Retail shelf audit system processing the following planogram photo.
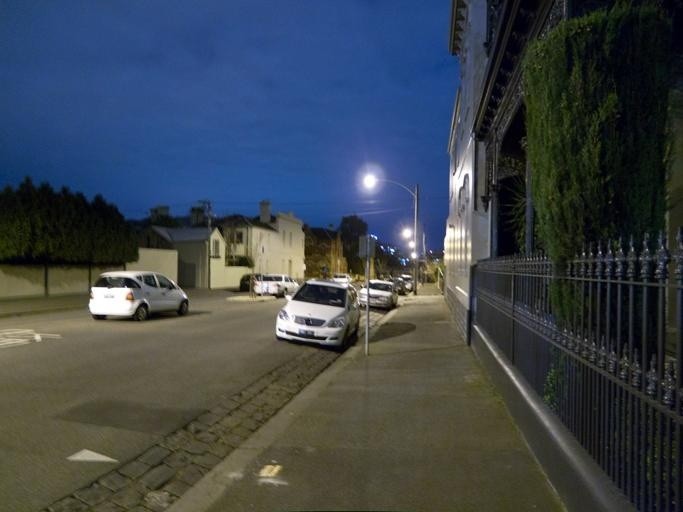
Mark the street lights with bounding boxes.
[364,173,419,294]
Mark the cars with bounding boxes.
[254,272,415,350]
[86,271,189,322]
